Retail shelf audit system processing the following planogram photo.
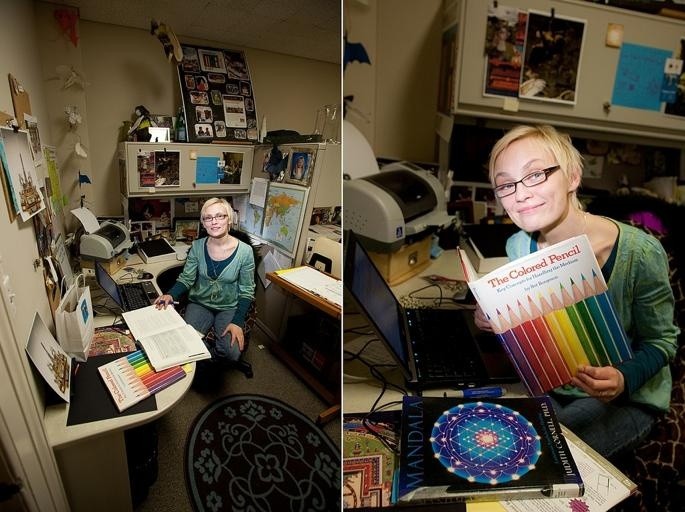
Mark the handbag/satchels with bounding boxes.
[55,274,96,363]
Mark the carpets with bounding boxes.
[182,393,341,512]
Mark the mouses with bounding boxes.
[451,289,476,305]
[137,272,154,280]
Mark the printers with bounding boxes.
[343,159,455,253]
[79,220,132,260]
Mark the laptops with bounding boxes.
[346,229,521,390]
[94,262,160,312]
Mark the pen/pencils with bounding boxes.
[443,386,508,397]
[158,300,180,305]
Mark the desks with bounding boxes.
[344,249,642,510]
[41,243,199,512]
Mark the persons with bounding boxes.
[292,155,305,180]
[191,92,205,104]
[197,126,205,136]
[205,128,211,136]
[139,158,152,173]
[488,25,514,62]
[196,110,205,122]
[154,196,258,379]
[127,104,159,142]
[486,122,681,458]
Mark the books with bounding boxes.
[120,303,212,373]
[456,234,636,398]
[397,394,585,505]
[96,349,186,414]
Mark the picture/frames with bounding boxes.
[148,126,171,143]
[284,146,317,186]
[171,215,202,241]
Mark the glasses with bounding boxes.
[494,166,560,199]
[202,214,228,221]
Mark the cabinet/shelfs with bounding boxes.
[241,143,341,352]
[259,265,344,426]
[436,0,685,144]
[119,140,255,198]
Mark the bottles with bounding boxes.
[175,105,186,142]
[260,114,267,144]
[307,104,339,144]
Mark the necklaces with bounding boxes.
[541,213,588,248]
[208,256,220,276]
